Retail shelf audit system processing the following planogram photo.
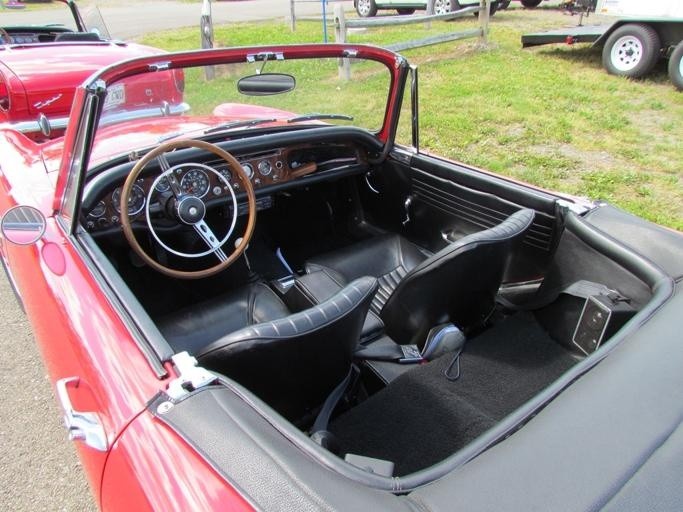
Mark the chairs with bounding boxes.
[147,273,379,416]
[302,206,538,354]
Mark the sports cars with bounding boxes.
[2,0,192,146]
[1,41,681,512]
